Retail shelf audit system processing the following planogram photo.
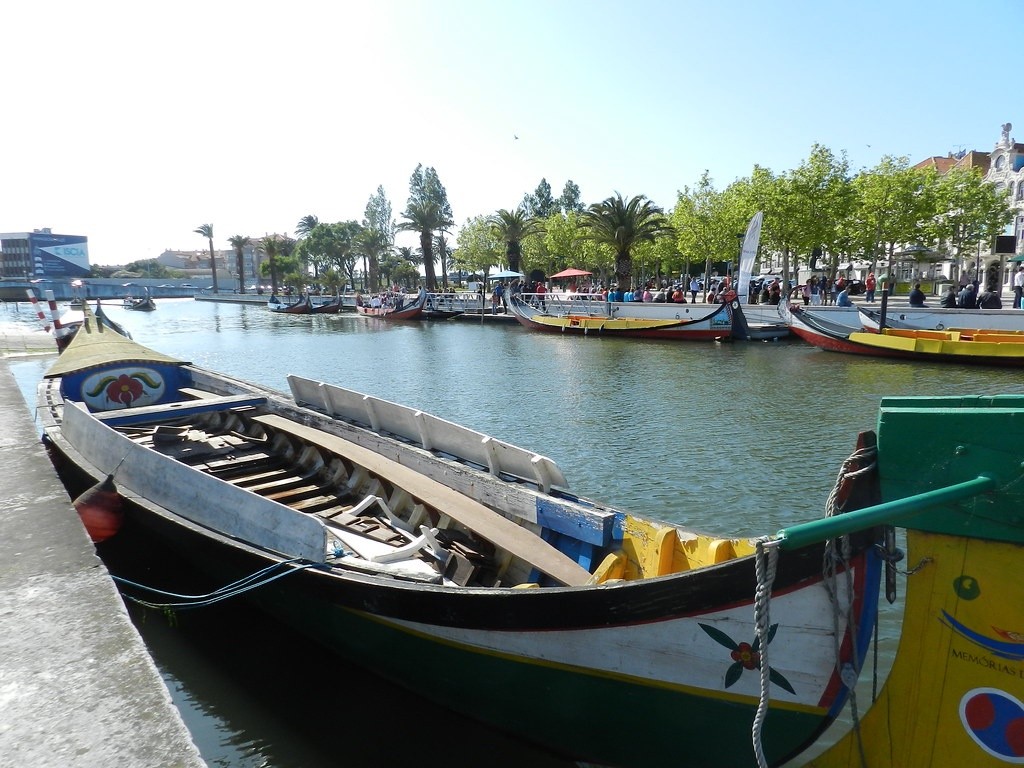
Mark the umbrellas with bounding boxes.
[487,269,524,285]
[550,267,593,280]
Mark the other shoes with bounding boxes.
[829,303,832,306]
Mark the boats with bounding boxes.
[504,287,751,349]
[855,305,1024,335]
[356,287,427,321]
[774,298,1024,363]
[31,300,1023,765]
[123,286,158,312]
[264,294,313,314]
[311,292,343,314]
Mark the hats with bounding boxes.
[966,284,974,289]
[869,273,875,277]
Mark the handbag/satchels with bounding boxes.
[708,294,714,301]
[800,287,807,291]
[683,299,687,303]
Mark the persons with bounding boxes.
[707,275,854,307]
[476,279,548,315]
[865,273,876,302]
[888,273,897,296]
[1012,261,1024,308]
[941,270,1002,309]
[909,283,929,308]
[357,284,455,309]
[561,274,699,304]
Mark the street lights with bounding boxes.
[546,257,559,289]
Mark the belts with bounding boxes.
[759,300,768,303]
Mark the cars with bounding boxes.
[124,282,139,287]
[30,278,52,283]
[159,283,193,288]
